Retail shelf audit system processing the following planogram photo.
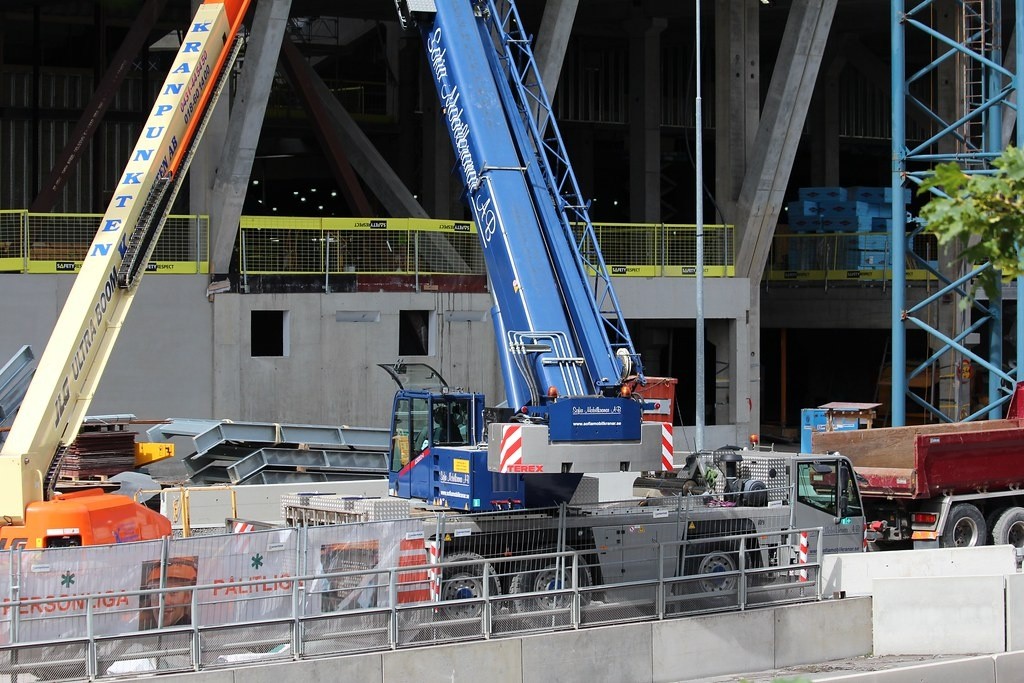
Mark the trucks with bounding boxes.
[810,376,1023,568]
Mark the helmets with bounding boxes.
[145,556,197,585]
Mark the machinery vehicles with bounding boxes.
[280,1,792,618]
[1,1,336,679]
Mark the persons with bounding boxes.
[138,556,197,630]
[434,406,465,448]
